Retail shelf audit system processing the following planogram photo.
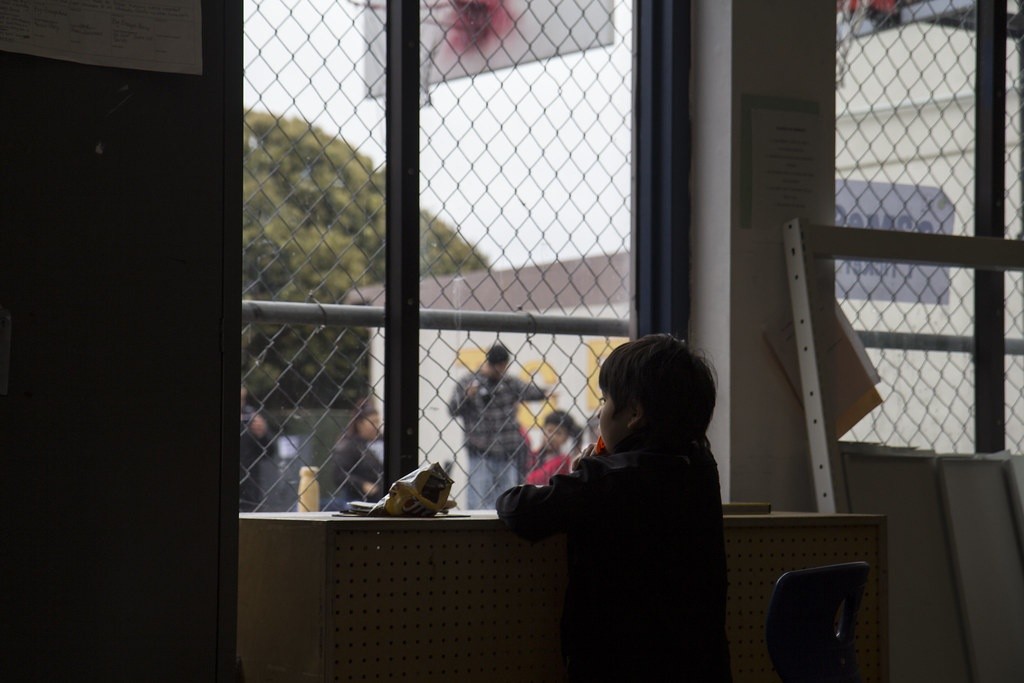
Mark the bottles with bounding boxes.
[296,467,319,512]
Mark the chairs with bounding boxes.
[764,560,871,683]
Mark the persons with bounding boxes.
[239,376,278,512]
[449,345,585,507]
[494,334,734,683]
[332,407,384,502]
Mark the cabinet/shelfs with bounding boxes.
[238,510,891,683]
[0,0,244,683]
